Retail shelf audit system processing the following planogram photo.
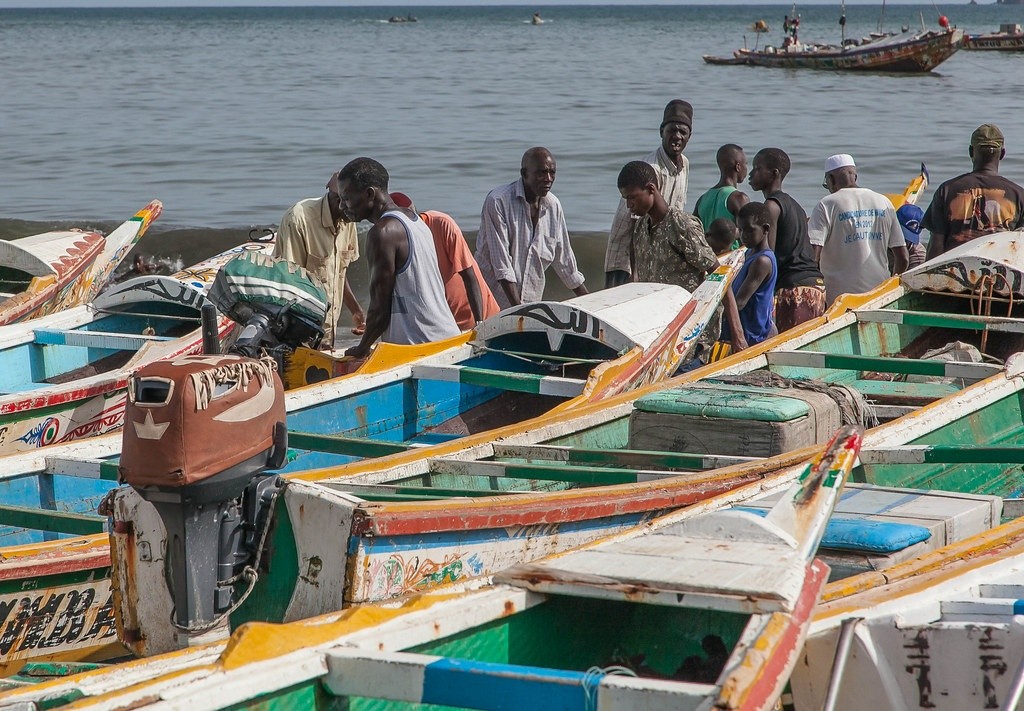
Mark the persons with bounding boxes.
[389,193,500,331]
[732,203,777,345]
[339,157,462,361]
[617,160,749,374]
[474,147,588,310]
[749,148,824,332]
[693,144,750,250]
[703,218,740,254]
[533,12,542,23]
[275,173,366,352]
[887,204,926,274]
[920,123,1024,262]
[604,99,692,288]
[809,154,909,310]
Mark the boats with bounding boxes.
[1,196,163,328]
[2,158,1024,711]
[738,0,969,74]
[522,19,553,25]
[389,15,421,25]
[702,53,755,72]
[958,24,1024,53]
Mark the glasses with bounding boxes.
[822,173,833,189]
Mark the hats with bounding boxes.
[388,191,414,210]
[824,153,855,172]
[895,204,926,244]
[661,100,693,132]
[971,123,1004,149]
